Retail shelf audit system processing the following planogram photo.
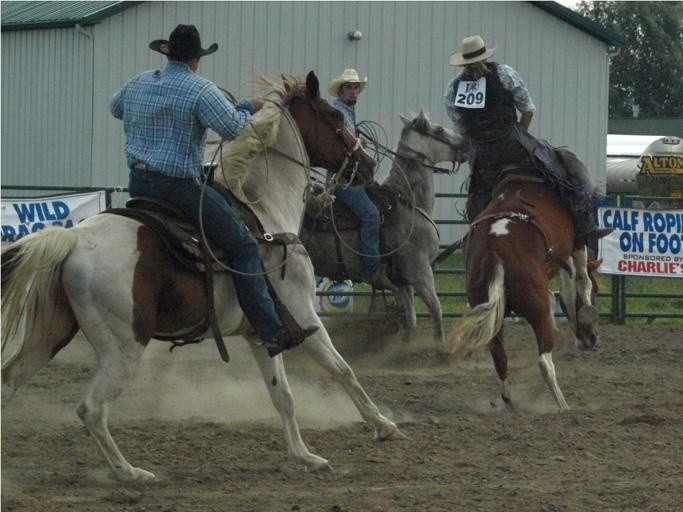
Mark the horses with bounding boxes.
[1,69,410,486]
[456,149,604,411]
[299,106,470,347]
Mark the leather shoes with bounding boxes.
[266,325,318,357]
[369,272,398,291]
[574,228,613,248]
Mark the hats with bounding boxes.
[150,24,218,57]
[448,35,496,66]
[328,69,367,97]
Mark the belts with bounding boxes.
[132,163,154,171]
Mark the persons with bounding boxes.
[107,24,321,359]
[443,34,616,251]
[325,68,399,293]
